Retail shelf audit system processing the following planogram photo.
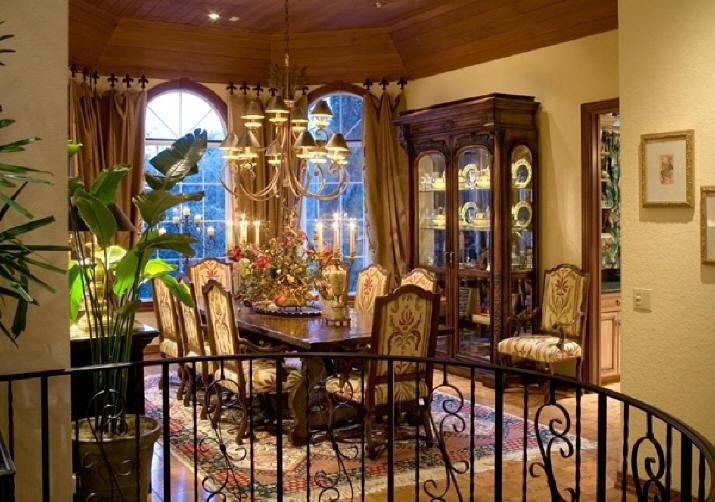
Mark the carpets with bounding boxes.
[143,372,598,500]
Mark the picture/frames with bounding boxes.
[700,185,715,265]
[640,128,695,210]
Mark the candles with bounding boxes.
[158,207,215,237]
[227,221,260,243]
[318,223,355,253]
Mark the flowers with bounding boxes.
[227,206,336,308]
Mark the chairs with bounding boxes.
[186,257,236,296]
[152,273,210,421]
[318,258,441,314]
[495,264,593,407]
[199,279,300,446]
[322,282,441,453]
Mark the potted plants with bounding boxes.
[65,126,209,500]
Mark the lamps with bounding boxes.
[216,1,354,202]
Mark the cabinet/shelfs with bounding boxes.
[392,93,542,390]
[599,124,620,384]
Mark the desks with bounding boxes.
[193,301,379,443]
